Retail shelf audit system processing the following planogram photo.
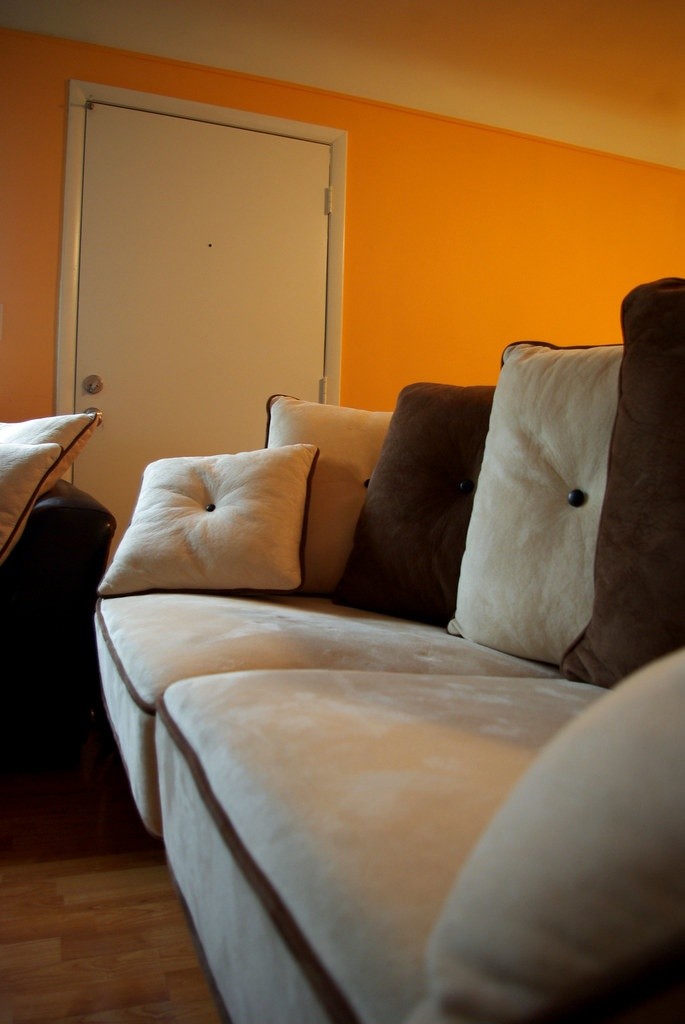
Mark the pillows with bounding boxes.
[265,394,393,598]
[0,413,104,566]
[448,341,624,668]
[333,382,495,627]
[555,276,685,690]
[96,443,321,597]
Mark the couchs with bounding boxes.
[95,597,685,1024]
[0,482,116,776]
